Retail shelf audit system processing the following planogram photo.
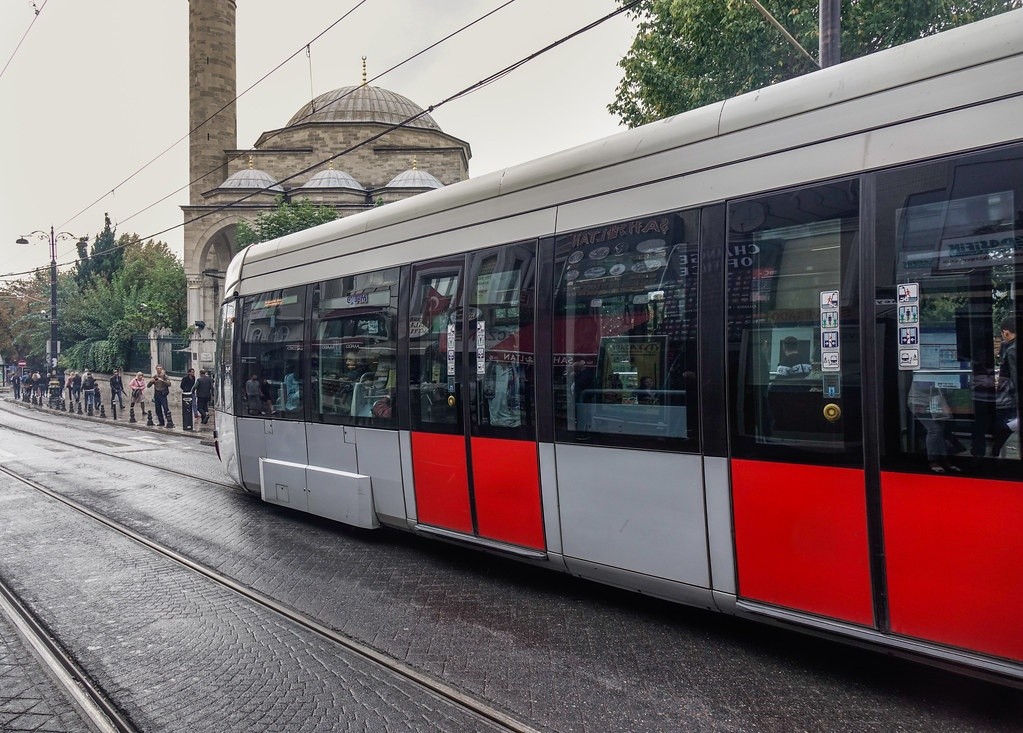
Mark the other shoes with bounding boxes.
[200,416,208,424]
[261,411,266,415]
[205,412,209,416]
[271,410,276,415]
[142,413,148,414]
[194,416,200,418]
[167,422,173,428]
[156,423,164,426]
[120,405,124,409]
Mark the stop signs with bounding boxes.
[19,359,27,369]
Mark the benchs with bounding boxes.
[575,387,688,440]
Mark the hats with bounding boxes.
[199,369,205,373]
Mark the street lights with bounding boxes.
[15,225,89,410]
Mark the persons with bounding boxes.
[129,372,147,414]
[334,318,621,426]
[10,370,101,410]
[632,376,659,405]
[180,369,214,424]
[769,336,811,399]
[147,364,171,426]
[284,362,306,414]
[908,317,1023,471]
[109,370,125,409]
[246,371,276,417]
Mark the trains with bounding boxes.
[213,5,1023,690]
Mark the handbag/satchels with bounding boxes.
[132,389,140,398]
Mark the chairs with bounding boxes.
[351,370,390,418]
